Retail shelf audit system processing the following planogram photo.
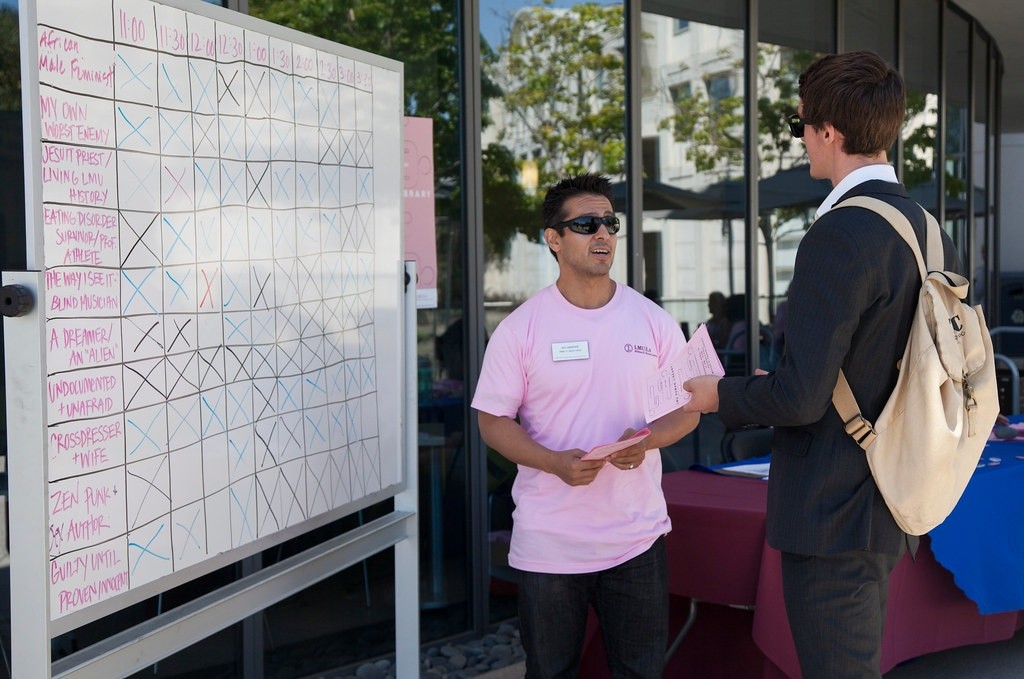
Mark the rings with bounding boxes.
[629,463,634,470]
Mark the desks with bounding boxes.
[578,414,1023,679]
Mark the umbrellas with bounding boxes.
[598,158,988,325]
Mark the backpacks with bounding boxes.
[805,200,1001,535]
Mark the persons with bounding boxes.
[434,314,490,392]
[682,50,960,678]
[645,285,785,379]
[470,173,703,679]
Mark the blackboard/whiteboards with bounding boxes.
[1,1,425,639]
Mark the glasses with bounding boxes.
[788,114,812,138]
[550,215,620,235]
